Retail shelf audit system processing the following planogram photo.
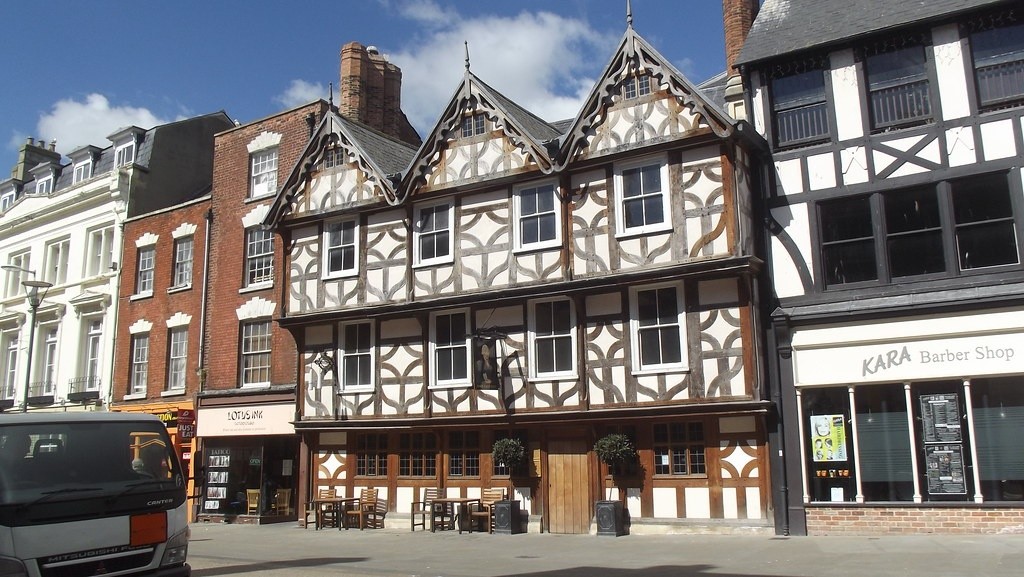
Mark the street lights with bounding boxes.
[21,281,54,412]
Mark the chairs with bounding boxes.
[246,488,260,515]
[411,488,446,533]
[470,489,504,534]
[270,489,291,515]
[304,489,336,530]
[346,488,378,531]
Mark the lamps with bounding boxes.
[315,347,339,374]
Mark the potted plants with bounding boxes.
[492,437,526,534]
[593,433,636,537]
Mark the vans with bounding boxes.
[0,412,192,577]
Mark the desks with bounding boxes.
[431,498,480,534]
[315,498,361,531]
